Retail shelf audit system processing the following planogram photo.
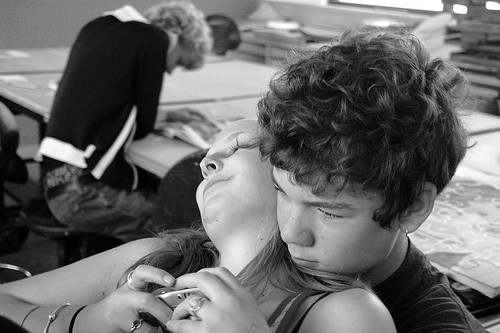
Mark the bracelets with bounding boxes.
[68,305,88,333]
[44,303,71,333]
[20,306,40,327]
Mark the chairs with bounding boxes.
[146,147,210,238]
[0,100,82,268]
[205,14,241,56]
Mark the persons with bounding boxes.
[0,119,398,333]
[31,0,214,242]
[237,24,488,333]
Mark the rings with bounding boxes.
[190,297,207,318]
[127,270,146,289]
[131,318,143,333]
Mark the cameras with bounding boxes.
[145,287,209,331]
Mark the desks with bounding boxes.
[0,46,500,318]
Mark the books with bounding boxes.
[156,120,225,150]
[452,50,500,116]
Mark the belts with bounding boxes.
[44,172,98,200]
